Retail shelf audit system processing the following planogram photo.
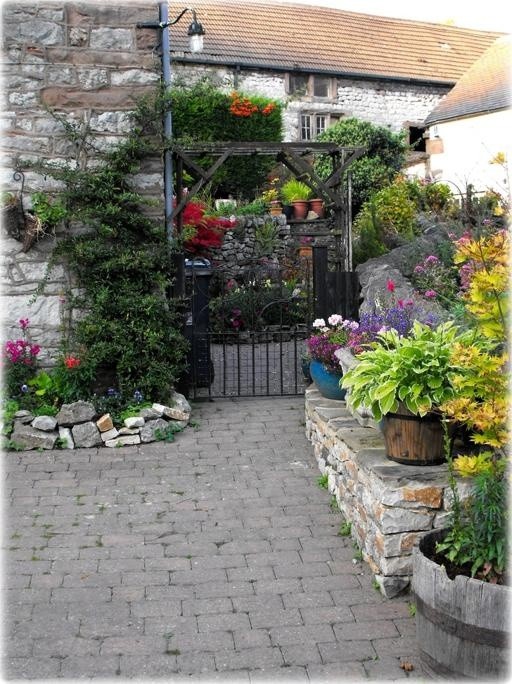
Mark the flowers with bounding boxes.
[344,279,439,357]
[304,316,359,375]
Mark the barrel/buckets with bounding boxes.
[412,525,511,683]
[381,399,454,466]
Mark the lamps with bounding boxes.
[136,7,206,55]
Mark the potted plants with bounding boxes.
[413,412,511,684]
[338,320,500,465]
[269,176,327,220]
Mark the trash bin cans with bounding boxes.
[184,257,215,387]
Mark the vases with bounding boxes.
[309,360,345,400]
[334,349,382,431]
